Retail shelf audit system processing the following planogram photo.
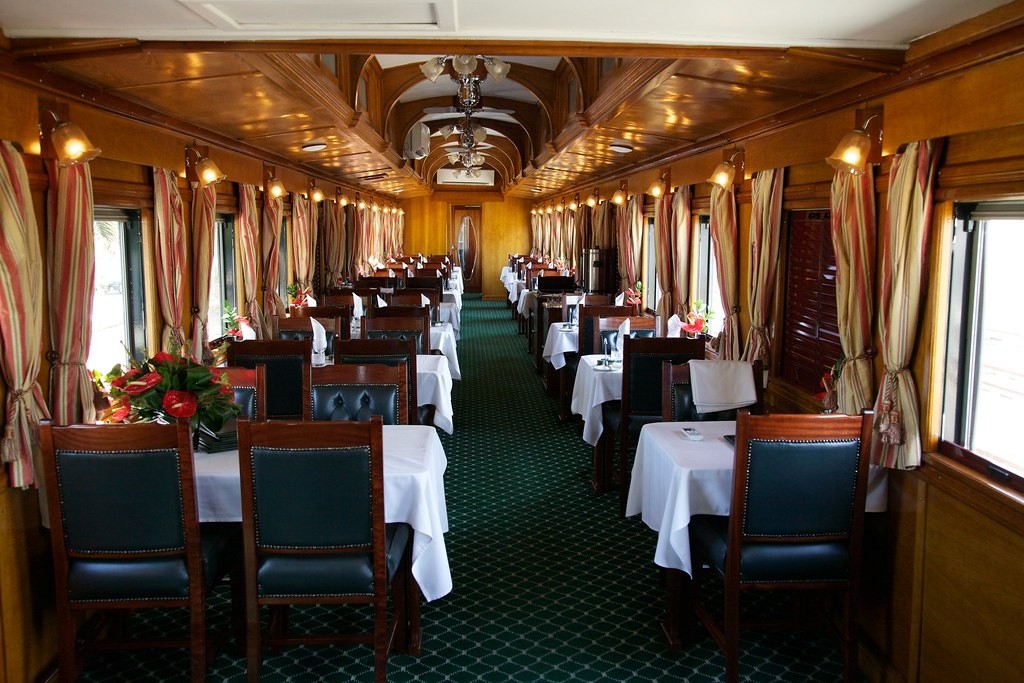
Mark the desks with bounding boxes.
[208,350,456,435]
[521,288,609,354]
[321,299,460,342]
[73,417,453,655]
[446,268,464,310]
[548,303,675,418]
[568,350,758,492]
[624,421,895,649]
[282,318,464,381]
[500,266,526,321]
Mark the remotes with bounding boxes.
[681,428,703,441]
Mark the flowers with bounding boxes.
[809,353,846,408]
[89,340,245,450]
[623,277,647,309]
[680,296,713,340]
[220,299,259,340]
[286,280,314,308]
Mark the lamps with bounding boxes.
[420,53,517,174]
[36,98,101,167]
[184,145,228,187]
[647,164,674,198]
[823,103,884,173]
[706,146,750,190]
[262,162,291,199]
[306,180,404,217]
[530,173,629,219]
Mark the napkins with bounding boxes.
[239,251,451,367]
[514,253,681,364]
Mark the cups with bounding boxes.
[610,345,623,368]
[350,316,359,332]
[312,348,325,366]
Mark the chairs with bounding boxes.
[509,256,883,683]
[28,254,454,683]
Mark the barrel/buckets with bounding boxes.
[582,249,605,293]
